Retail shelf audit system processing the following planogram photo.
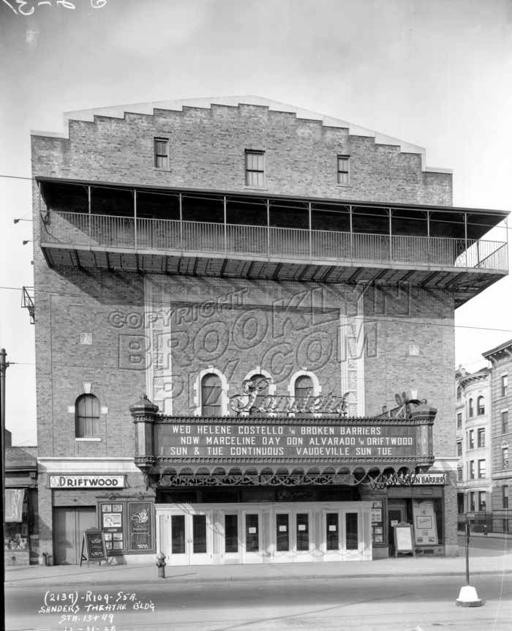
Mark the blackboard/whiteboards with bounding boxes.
[394,525,415,552]
[84,529,108,561]
